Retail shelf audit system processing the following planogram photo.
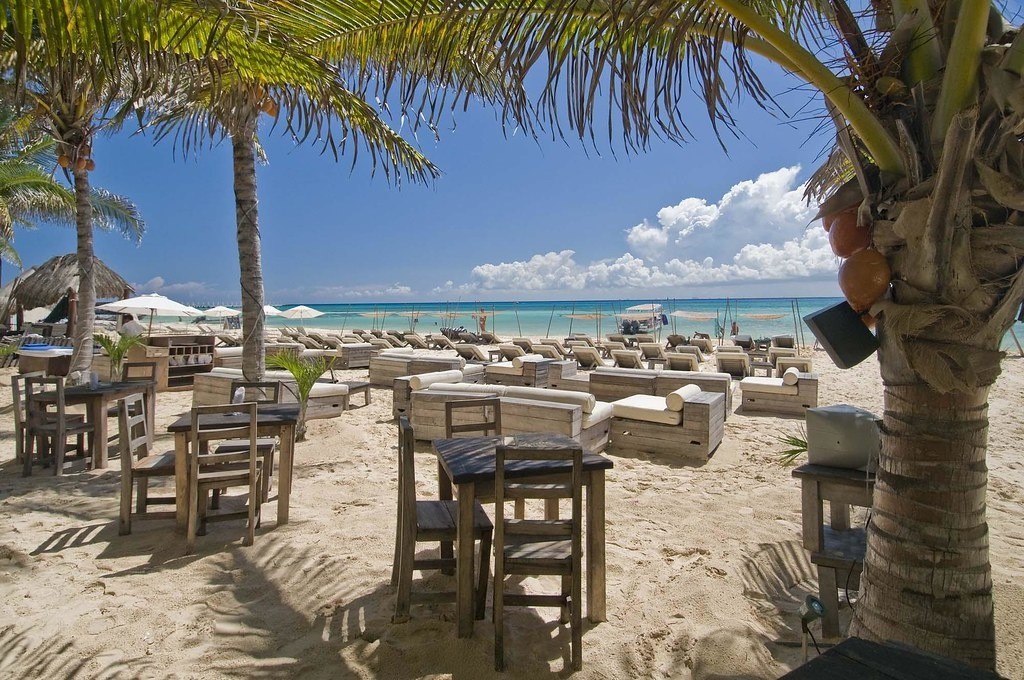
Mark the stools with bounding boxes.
[793,461,888,557]
[811,522,877,632]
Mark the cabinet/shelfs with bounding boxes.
[127,337,217,392]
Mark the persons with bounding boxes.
[622,320,640,336]
[118,314,143,359]
[729,322,739,338]
[479,308,486,333]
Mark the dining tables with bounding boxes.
[431,431,615,633]
[165,405,303,536]
[32,379,158,467]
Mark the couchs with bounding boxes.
[739,366,818,418]
[487,353,549,388]
[194,365,349,418]
[587,364,733,418]
[608,383,726,463]
[370,347,485,386]
[214,341,372,368]
[394,369,615,457]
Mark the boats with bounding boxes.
[618,303,664,332]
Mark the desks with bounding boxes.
[755,337,771,351]
[750,361,774,377]
[646,357,668,369]
[596,345,608,358]
[748,349,768,362]
[487,349,505,362]
[564,337,574,347]
[628,337,640,348]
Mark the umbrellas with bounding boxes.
[204,306,241,334]
[265,305,325,334]
[95,290,207,345]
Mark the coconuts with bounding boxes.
[821,205,889,315]
[250,83,278,116]
[59,144,96,171]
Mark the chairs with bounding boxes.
[776,356,813,376]
[691,338,713,352]
[640,343,667,363]
[769,348,797,368]
[10,370,86,462]
[771,335,795,349]
[636,333,655,346]
[431,332,455,350]
[439,397,505,567]
[458,332,480,343]
[481,333,503,343]
[717,346,744,354]
[611,350,645,368]
[532,344,565,360]
[334,376,373,407]
[676,346,705,362]
[387,413,498,639]
[606,334,628,345]
[568,341,591,349]
[455,344,487,362]
[734,335,754,349]
[539,338,576,361]
[547,360,590,391]
[183,400,263,554]
[498,344,526,360]
[576,335,594,347]
[20,376,97,476]
[513,337,534,353]
[489,446,589,673]
[208,380,286,512]
[218,327,429,349]
[107,360,158,457]
[605,341,626,356]
[667,353,699,372]
[716,353,750,380]
[571,345,608,368]
[115,393,209,540]
[667,334,688,346]
